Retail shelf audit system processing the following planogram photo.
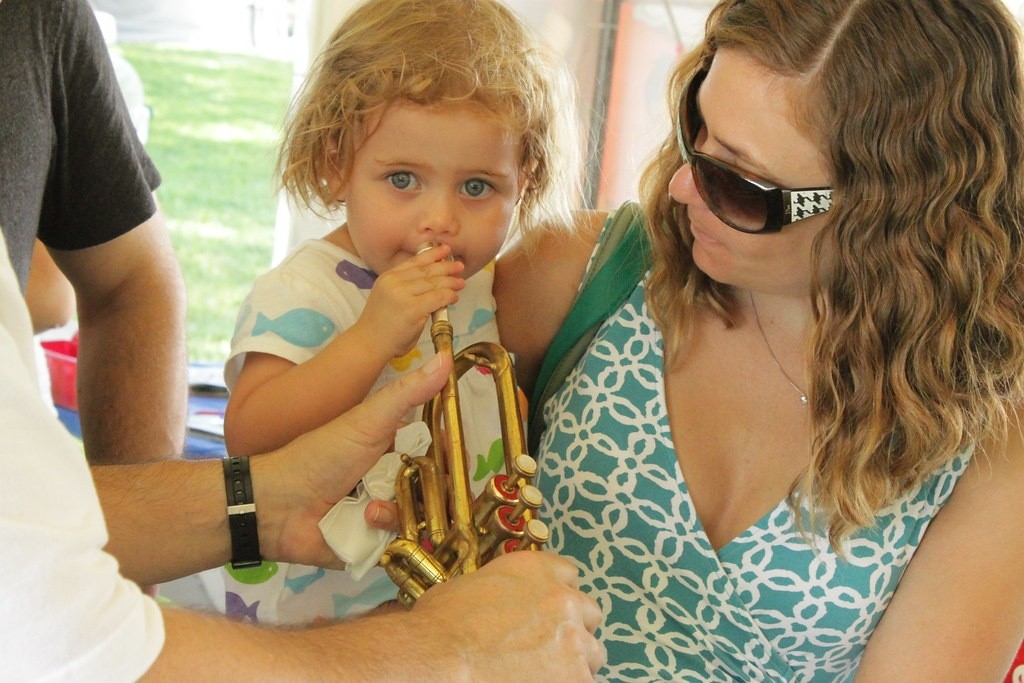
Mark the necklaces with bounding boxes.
[749,291,808,407]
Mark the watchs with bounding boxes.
[220,455,263,570]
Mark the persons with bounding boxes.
[0,232,608,683]
[0,0,191,595]
[493,0,1024,683]
[223,0,557,625]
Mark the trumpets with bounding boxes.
[380,241,550,611]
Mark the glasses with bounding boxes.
[674,55,837,235]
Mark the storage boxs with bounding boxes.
[40,332,81,413]
